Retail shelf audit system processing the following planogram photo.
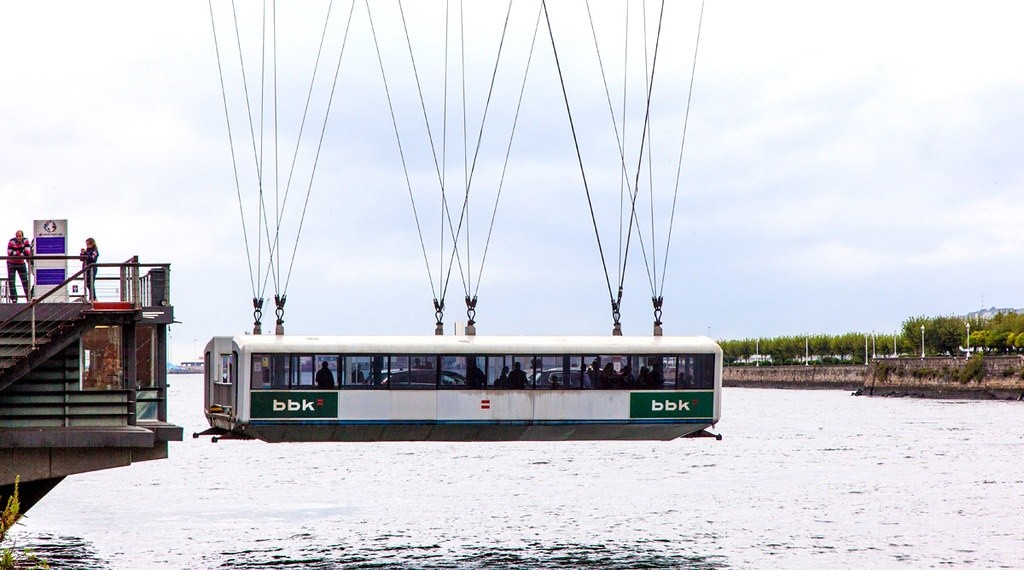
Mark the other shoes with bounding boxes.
[12,299,17,302]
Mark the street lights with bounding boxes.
[965,322,971,357]
[805,337,809,366]
[921,325,926,358]
[893,330,898,356]
[756,339,760,367]
[872,333,876,358]
[864,333,869,366]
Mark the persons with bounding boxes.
[466,359,693,390]
[315,357,433,389]
[7,230,37,303]
[80,238,99,303]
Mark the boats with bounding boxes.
[190,309,723,444]
